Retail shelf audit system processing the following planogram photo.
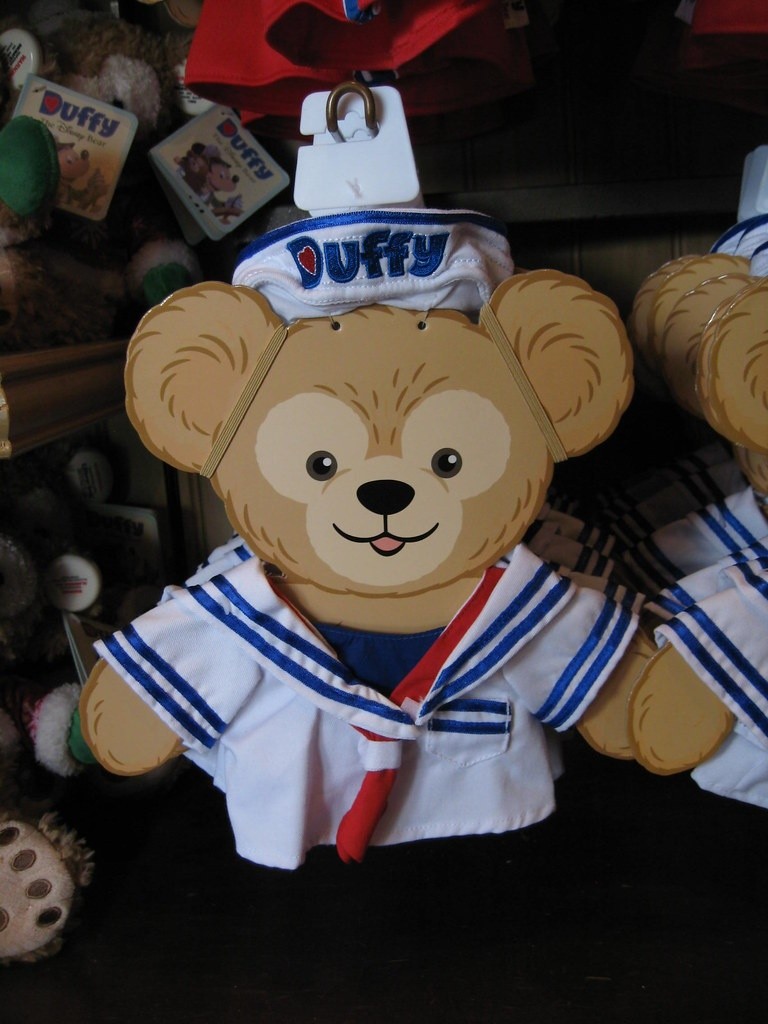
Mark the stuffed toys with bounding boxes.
[0,476,189,969]
[0,9,222,352]
